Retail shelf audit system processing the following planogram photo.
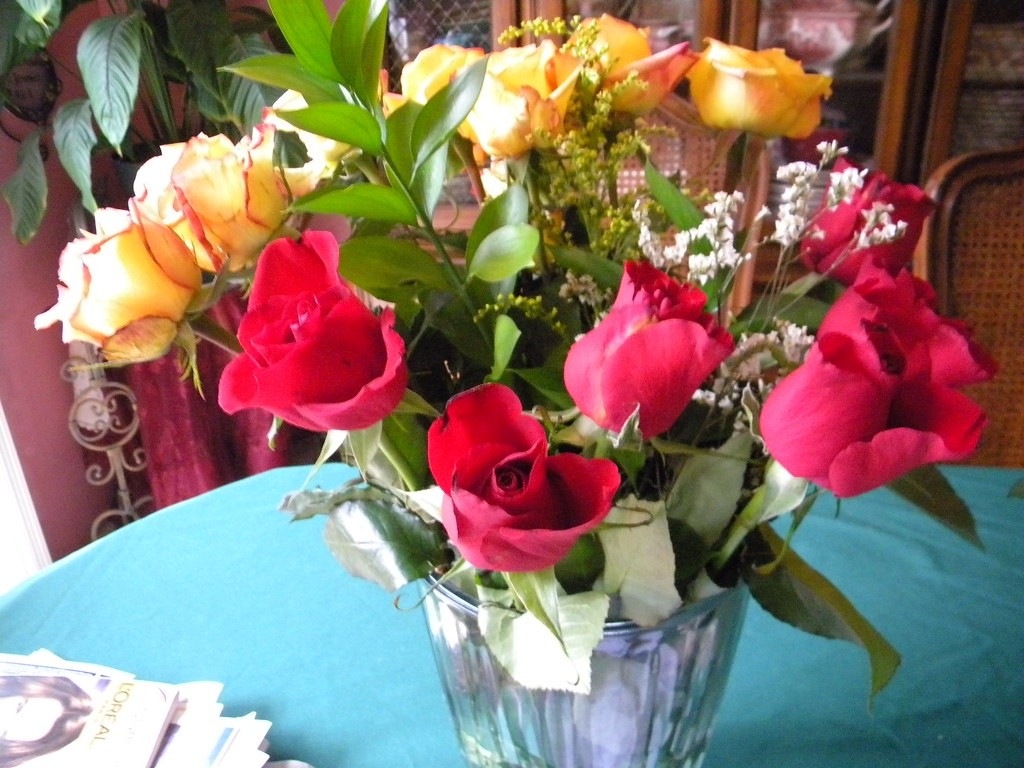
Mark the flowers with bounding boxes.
[28,0,997,703]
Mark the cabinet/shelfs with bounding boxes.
[379,0,943,383]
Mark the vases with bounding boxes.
[418,572,749,768]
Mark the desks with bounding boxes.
[0,461,1024,768]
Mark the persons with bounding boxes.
[0,672,90,768]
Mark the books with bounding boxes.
[0,646,274,767]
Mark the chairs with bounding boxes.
[911,145,1024,469]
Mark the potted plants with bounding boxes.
[0,0,295,247]
